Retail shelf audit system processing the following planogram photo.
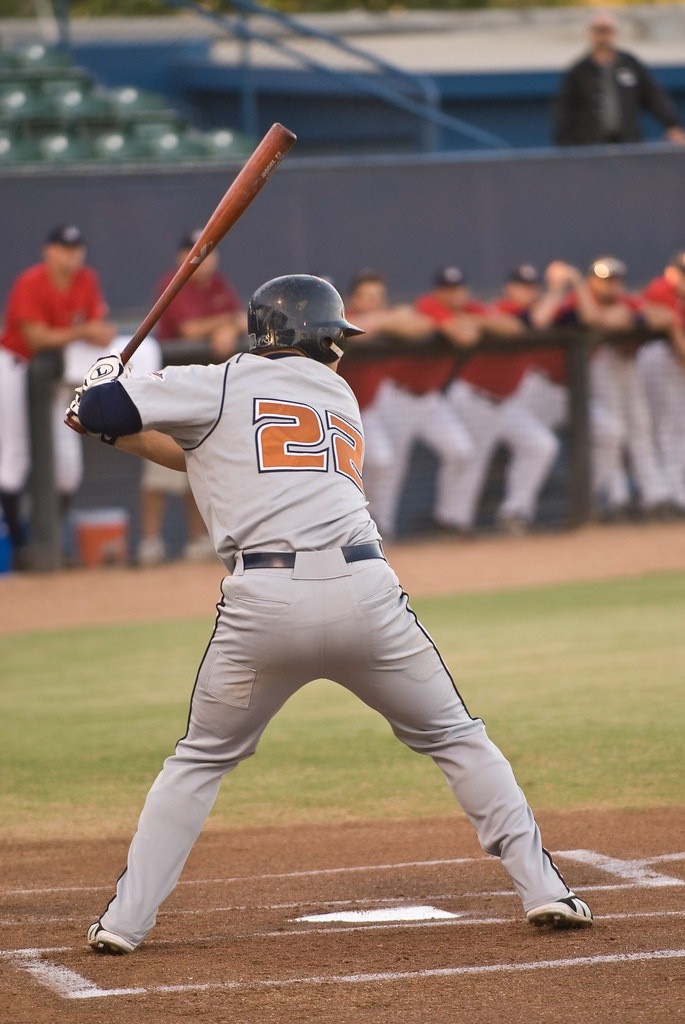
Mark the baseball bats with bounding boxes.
[64,119,299,434]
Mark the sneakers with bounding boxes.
[526,891,593,928]
[86,921,135,954]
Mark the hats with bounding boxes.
[435,265,465,285]
[45,223,85,246]
[589,258,625,276]
[169,222,205,247]
[509,261,542,284]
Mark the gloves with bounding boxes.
[82,349,134,392]
[65,386,119,445]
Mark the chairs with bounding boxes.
[0,42,260,164]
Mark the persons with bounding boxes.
[553,15,685,146]
[340,250,685,539]
[63,274,592,951]
[0,224,117,571]
[137,230,250,563]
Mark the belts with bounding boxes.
[242,542,387,569]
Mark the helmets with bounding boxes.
[247,274,367,365]
